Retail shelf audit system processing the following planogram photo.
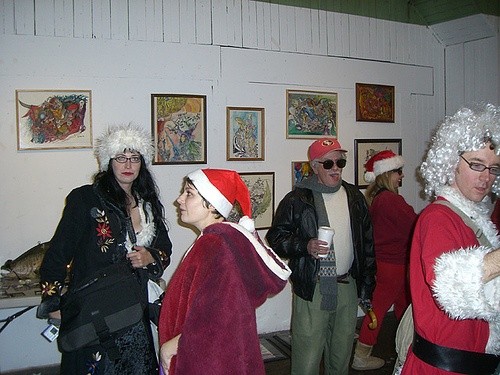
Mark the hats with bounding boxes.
[186,169,256,234]
[94,122,155,174]
[308,137,347,161]
[415,101,500,200]
[364,150,405,184]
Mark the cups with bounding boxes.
[316,225,335,258]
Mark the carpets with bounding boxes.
[257,336,291,363]
[273,331,293,350]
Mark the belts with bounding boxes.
[337,262,355,284]
[412,330,500,375]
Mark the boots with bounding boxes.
[351,340,385,370]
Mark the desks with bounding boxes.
[0,276,67,334]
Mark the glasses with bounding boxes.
[459,155,500,177]
[315,159,347,170]
[392,169,402,176]
[110,157,142,163]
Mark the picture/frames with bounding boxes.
[354,139,403,189]
[355,83,396,124]
[285,89,339,139]
[150,93,208,165]
[238,172,276,230]
[15,89,93,151]
[226,106,266,161]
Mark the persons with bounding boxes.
[155,167,292,375]
[265,136,377,375]
[390,102,500,375]
[352,147,422,371]
[34,120,171,375]
